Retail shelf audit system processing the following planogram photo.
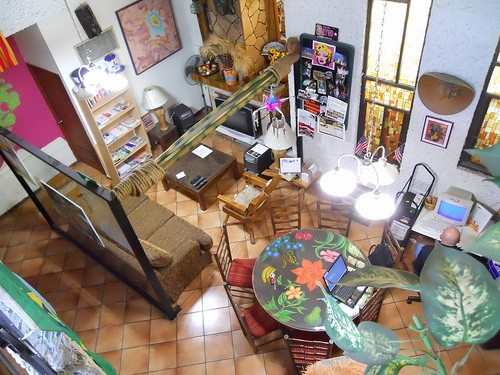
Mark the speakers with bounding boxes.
[169,103,194,131]
[243,142,273,175]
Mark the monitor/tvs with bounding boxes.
[433,186,474,227]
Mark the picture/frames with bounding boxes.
[115,0,182,75]
[420,116,454,148]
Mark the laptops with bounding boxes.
[323,254,368,308]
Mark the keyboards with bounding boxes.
[420,218,461,236]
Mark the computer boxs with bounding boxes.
[388,215,413,248]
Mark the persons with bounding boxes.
[412,226,464,276]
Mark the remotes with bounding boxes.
[191,175,206,189]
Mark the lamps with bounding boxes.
[142,86,171,131]
[321,0,399,220]
[253,85,296,151]
[64,0,127,99]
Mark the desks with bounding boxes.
[252,228,375,332]
[270,157,319,190]
[162,144,241,212]
[409,203,485,258]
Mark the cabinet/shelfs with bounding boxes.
[80,75,151,181]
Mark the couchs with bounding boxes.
[66,189,213,303]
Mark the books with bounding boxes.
[96,100,129,127]
[103,117,151,175]
[87,88,110,109]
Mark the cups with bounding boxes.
[302,173,308,183]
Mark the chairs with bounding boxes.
[214,172,406,374]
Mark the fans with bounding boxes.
[184,54,209,116]
